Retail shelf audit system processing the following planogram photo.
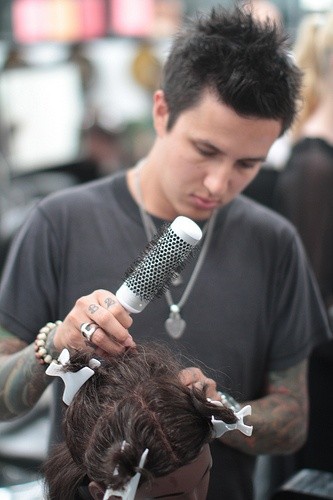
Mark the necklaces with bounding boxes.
[134,158,218,338]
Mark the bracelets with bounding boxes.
[33,320,62,366]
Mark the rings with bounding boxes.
[80,322,101,343]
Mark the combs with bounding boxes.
[115,216,203,315]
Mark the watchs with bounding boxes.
[219,391,240,413]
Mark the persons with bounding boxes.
[43,344,239,500]
[0,0,333,500]
